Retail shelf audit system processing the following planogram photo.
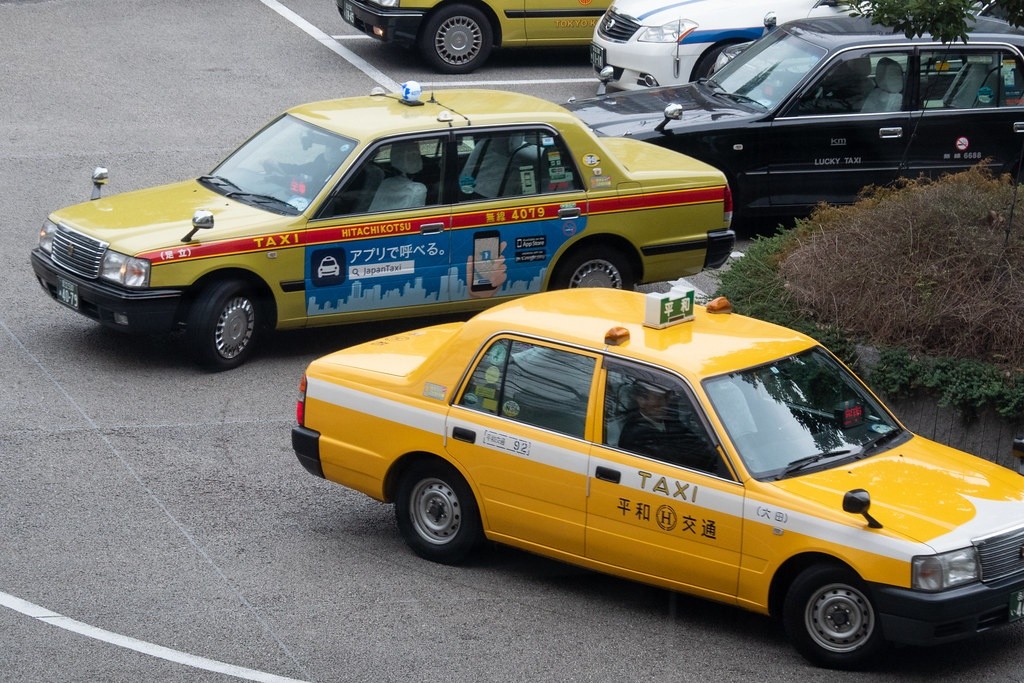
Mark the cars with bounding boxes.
[31,79,737,371]
[589,0,877,93]
[291,285,1024,670]
[560,14,1023,239]
[338,0,615,75]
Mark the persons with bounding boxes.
[268,145,361,217]
[616,379,706,472]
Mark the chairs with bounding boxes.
[478,345,761,473]
[338,131,546,213]
[830,54,1002,111]
